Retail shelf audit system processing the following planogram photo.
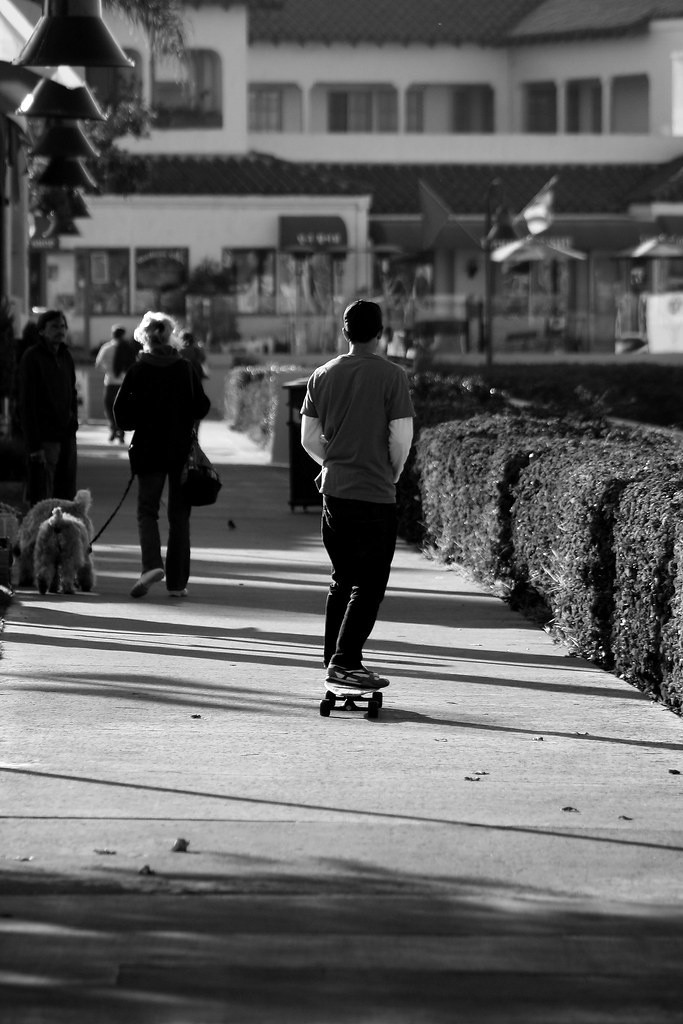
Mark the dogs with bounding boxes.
[1,490,94,594]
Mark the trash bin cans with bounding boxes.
[283,377,322,513]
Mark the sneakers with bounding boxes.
[322,662,390,688]
[167,588,188,597]
[130,568,164,598]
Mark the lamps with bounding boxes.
[10,0,136,239]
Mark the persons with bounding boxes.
[0,311,80,509]
[94,310,211,597]
[301,300,417,688]
[93,326,208,445]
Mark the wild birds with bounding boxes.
[228,520,236,530]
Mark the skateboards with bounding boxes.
[320,680,383,719]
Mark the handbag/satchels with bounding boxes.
[174,432,222,507]
